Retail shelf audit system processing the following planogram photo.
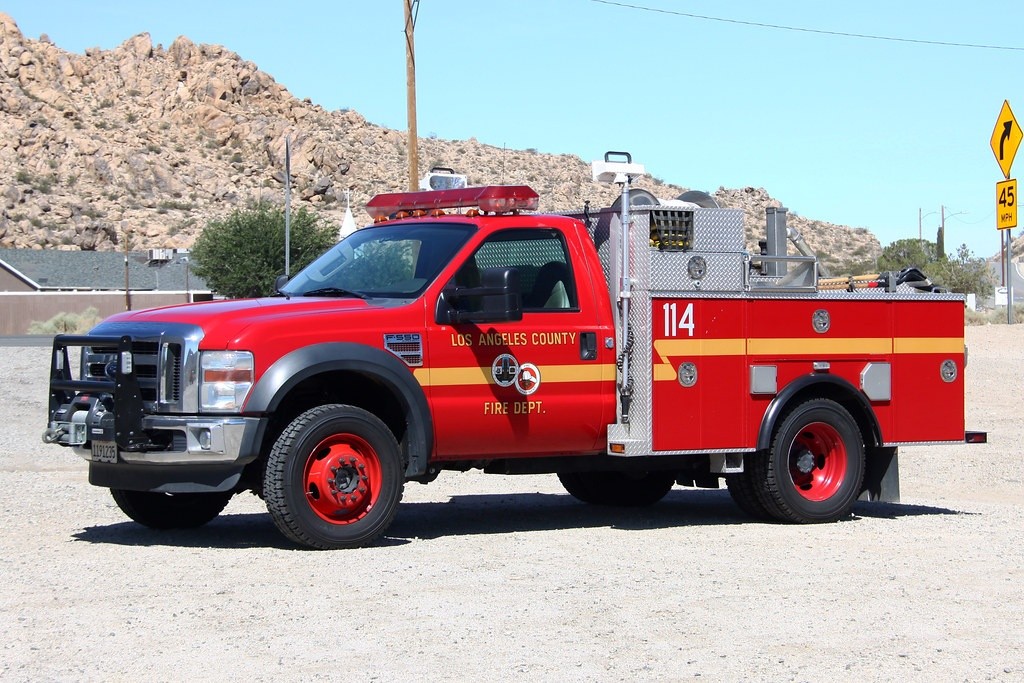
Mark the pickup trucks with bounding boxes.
[41,152,989,551]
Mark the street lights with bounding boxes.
[942,211,968,254]
[919,212,939,241]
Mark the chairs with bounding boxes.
[529,262,572,308]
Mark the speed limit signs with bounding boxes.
[996,178,1017,229]
